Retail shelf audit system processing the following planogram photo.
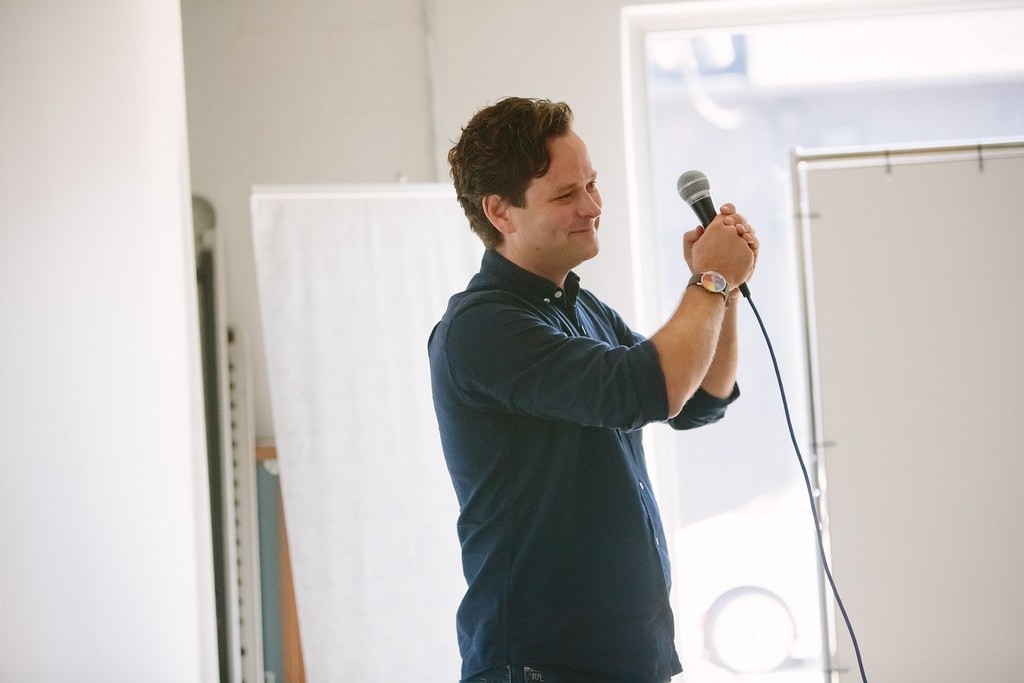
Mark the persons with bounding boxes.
[426,97,760,683]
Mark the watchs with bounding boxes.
[687,270,729,303]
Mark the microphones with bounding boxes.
[677,171,751,298]
